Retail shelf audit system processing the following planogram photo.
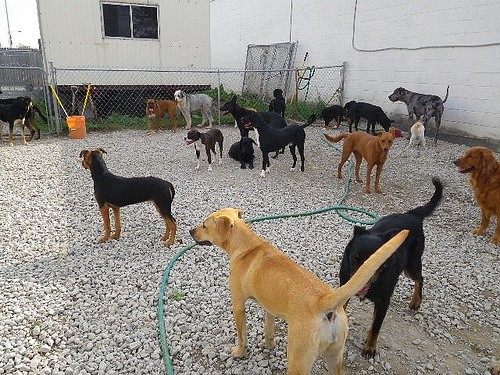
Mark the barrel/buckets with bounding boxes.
[66,116,86,139]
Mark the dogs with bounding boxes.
[78,147,178,249]
[267,88,286,119]
[240,110,317,178]
[387,85,450,148]
[321,130,394,195]
[319,104,343,129]
[453,144,500,246]
[0,90,48,143]
[334,175,447,361]
[341,99,358,125]
[345,101,396,137]
[187,208,412,375]
[219,94,288,139]
[143,98,178,135]
[182,128,225,172]
[0,96,33,148]
[407,120,427,150]
[173,90,215,129]
[227,136,258,170]
